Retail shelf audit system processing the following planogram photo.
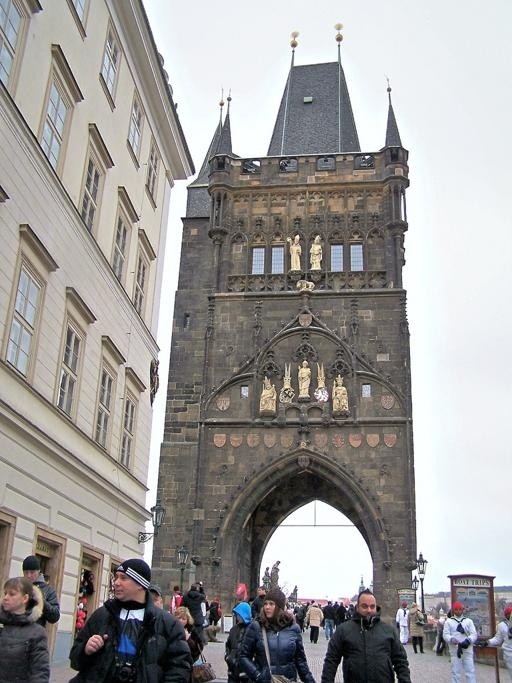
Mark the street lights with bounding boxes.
[177,545,189,594]
[416,553,429,623]
[411,574,419,607]
[263,577,271,590]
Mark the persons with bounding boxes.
[261,376,276,410]
[298,360,311,398]
[69,559,511,682]
[309,235,323,270]
[332,374,348,411]
[1,555,60,683]
[287,234,301,270]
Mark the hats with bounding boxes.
[22,556,41,570]
[148,584,163,598]
[452,602,463,608]
[116,558,151,591]
[503,608,512,616]
[263,589,286,611]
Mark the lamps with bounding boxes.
[137,498,165,545]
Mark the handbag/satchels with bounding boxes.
[270,674,301,683]
[189,662,217,683]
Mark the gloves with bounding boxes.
[474,640,489,649]
[456,643,463,659]
[460,638,470,649]
